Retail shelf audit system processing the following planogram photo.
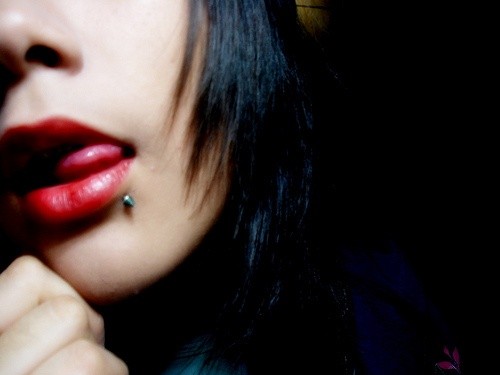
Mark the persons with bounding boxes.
[1,0,467,375]
